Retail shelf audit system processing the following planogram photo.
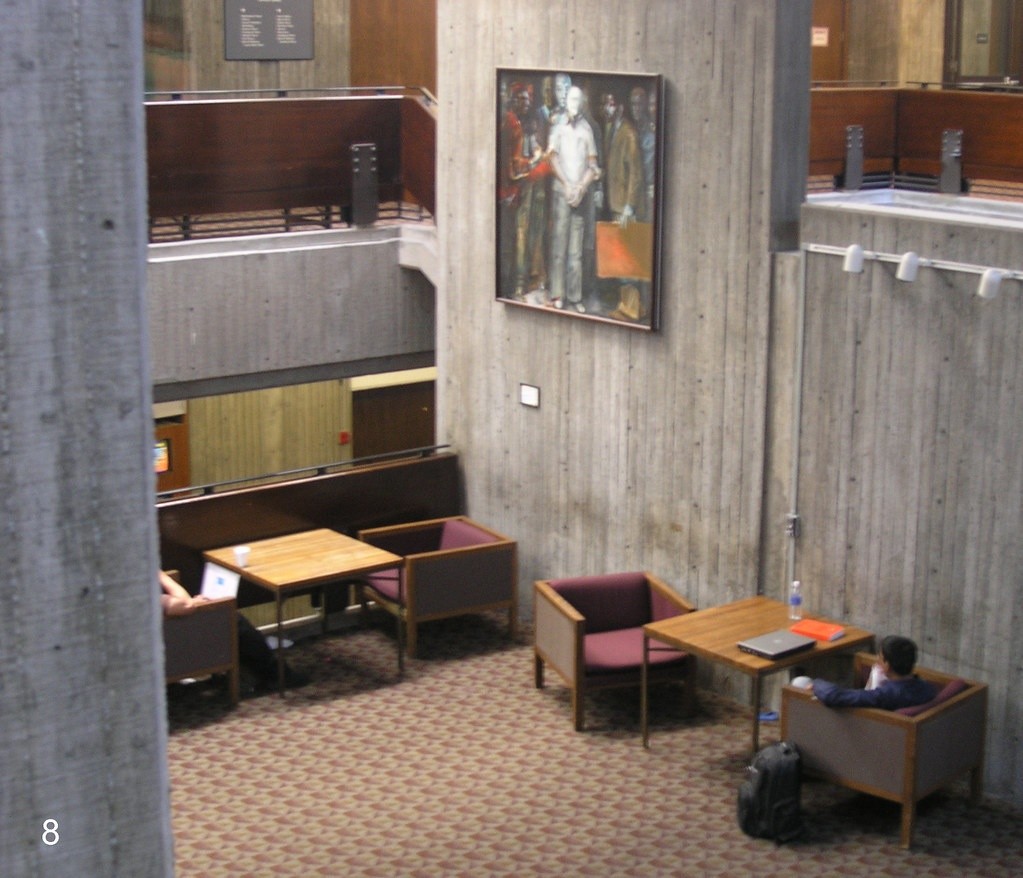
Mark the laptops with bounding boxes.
[201,561,240,603]
[737,628,816,662]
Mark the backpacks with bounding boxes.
[736,740,807,845]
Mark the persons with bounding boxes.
[159,568,310,686]
[807,634,936,708]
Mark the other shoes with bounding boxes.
[266,673,309,690]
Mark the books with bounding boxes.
[791,619,846,642]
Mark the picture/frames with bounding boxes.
[491,66,663,333]
[519,382,541,408]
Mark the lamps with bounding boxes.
[976,267,1014,300]
[841,242,865,276]
[894,251,920,282]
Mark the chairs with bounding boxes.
[359,517,519,660]
[533,570,697,731]
[158,568,239,699]
[779,650,990,850]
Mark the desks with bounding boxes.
[204,528,406,699]
[642,595,875,753]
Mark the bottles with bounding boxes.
[790,580,801,620]
